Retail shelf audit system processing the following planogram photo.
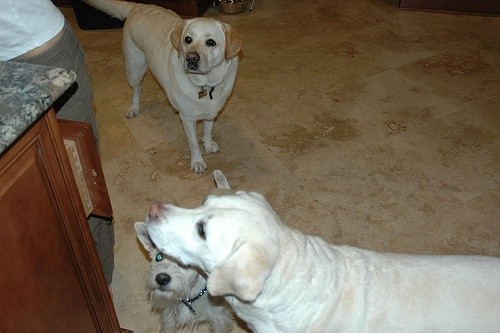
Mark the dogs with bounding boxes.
[85,0,243,176]
[132,169,235,333]
[145,188,500,332]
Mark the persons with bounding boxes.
[0,0,116,299]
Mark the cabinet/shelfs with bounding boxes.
[0,61,136,333]
